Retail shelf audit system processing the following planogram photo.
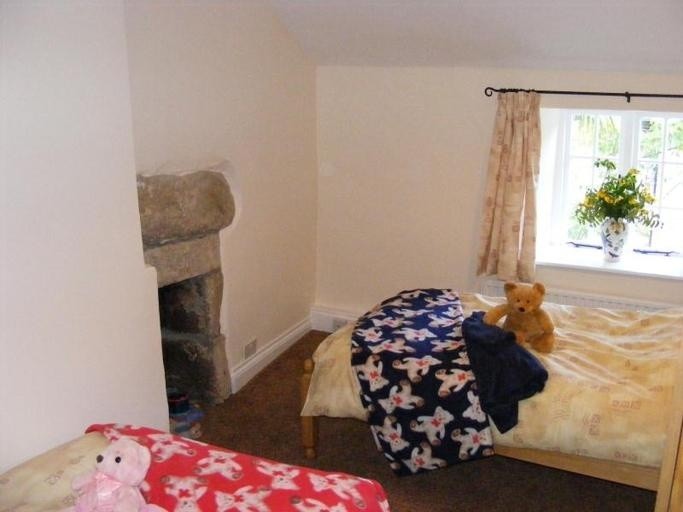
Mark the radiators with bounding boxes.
[483,279,676,311]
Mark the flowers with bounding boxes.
[573,159,661,228]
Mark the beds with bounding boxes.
[0,423,391,512]
[301,289,683,512]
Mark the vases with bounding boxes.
[600,217,628,263]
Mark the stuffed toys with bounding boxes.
[59,438,150,511]
[482,280,553,353]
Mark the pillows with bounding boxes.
[0,432,110,512]
